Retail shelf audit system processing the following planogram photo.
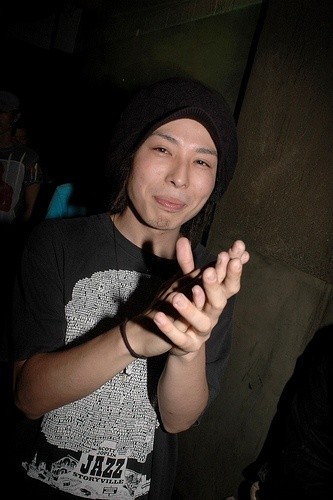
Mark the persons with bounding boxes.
[0,76,250,499]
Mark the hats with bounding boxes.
[110,78,239,211]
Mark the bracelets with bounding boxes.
[118,315,146,360]
